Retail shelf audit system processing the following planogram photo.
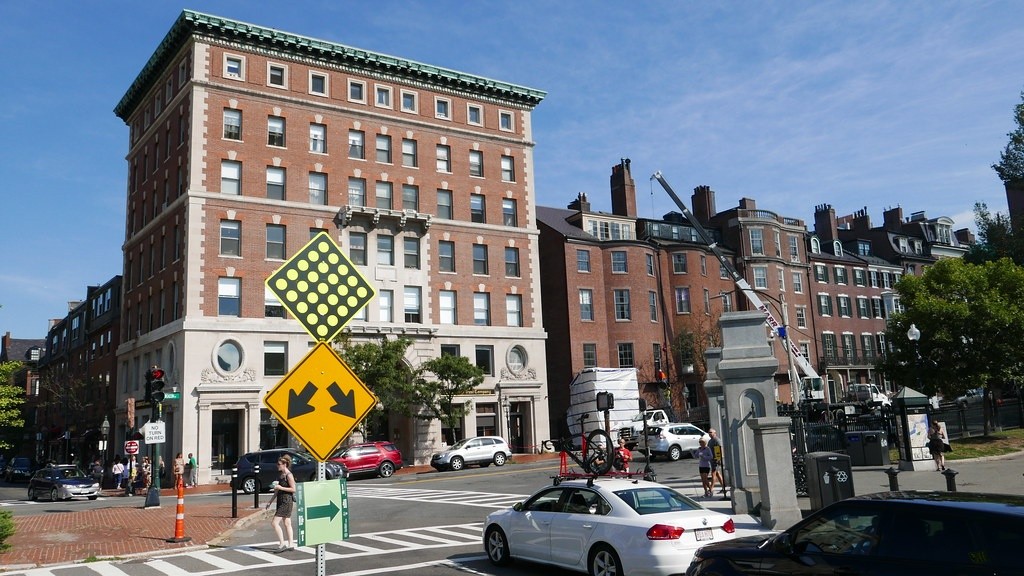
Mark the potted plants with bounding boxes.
[671,332,694,373]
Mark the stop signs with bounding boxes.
[124,441,139,455]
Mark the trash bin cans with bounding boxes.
[844,431,866,466]
[862,431,890,466]
[804,452,855,512]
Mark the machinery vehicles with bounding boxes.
[647,170,890,423]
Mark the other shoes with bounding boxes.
[718,490,725,494]
[708,489,711,494]
[186,486,193,488]
[274,545,286,553]
[117,487,122,489]
[708,493,713,497]
[940,464,945,470]
[936,467,940,472]
[194,485,198,488]
[701,494,708,498]
[285,546,294,551]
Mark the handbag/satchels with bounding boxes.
[613,451,624,469]
[121,472,124,478]
[293,496,297,503]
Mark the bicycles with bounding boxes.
[542,413,615,476]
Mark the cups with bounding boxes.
[699,453,703,460]
[273,480,279,490]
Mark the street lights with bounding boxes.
[99,415,114,490]
[906,323,925,395]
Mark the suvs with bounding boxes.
[429,435,514,472]
[329,441,404,479]
[639,422,713,463]
[4,456,34,483]
[230,449,351,495]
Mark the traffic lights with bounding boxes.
[659,380,667,391]
[596,390,614,411]
[132,431,144,440]
[150,366,166,405]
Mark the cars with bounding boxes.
[683,486,1024,576]
[481,476,735,576]
[28,464,100,501]
[955,389,984,408]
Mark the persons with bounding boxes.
[65,471,76,478]
[594,444,613,471]
[707,428,727,494]
[112,459,124,489]
[690,437,713,498]
[126,454,137,483]
[613,438,633,478]
[186,453,197,488]
[71,455,79,465]
[173,452,185,489]
[121,457,129,488]
[88,454,104,487]
[265,454,296,553]
[928,419,945,471]
[112,454,121,489]
[135,455,165,494]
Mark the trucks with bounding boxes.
[616,409,711,449]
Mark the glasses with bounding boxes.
[277,463,284,466]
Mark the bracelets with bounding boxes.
[267,503,271,506]
